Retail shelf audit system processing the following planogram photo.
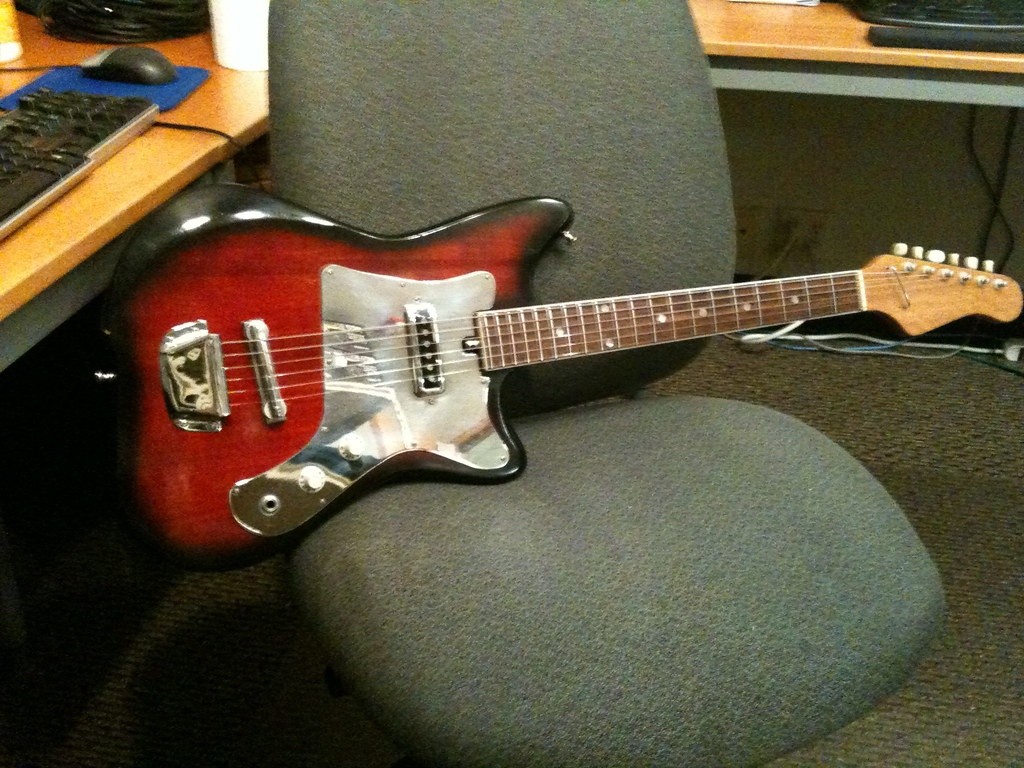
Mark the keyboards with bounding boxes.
[0,86,161,242]
[853,0,1024,33]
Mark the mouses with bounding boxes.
[80,45,177,85]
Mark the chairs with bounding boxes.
[266,0,948,768]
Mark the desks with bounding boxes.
[0,0,266,391]
[687,0,1024,108]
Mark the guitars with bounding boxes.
[91,178,1023,572]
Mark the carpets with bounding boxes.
[0,296,1024,768]
